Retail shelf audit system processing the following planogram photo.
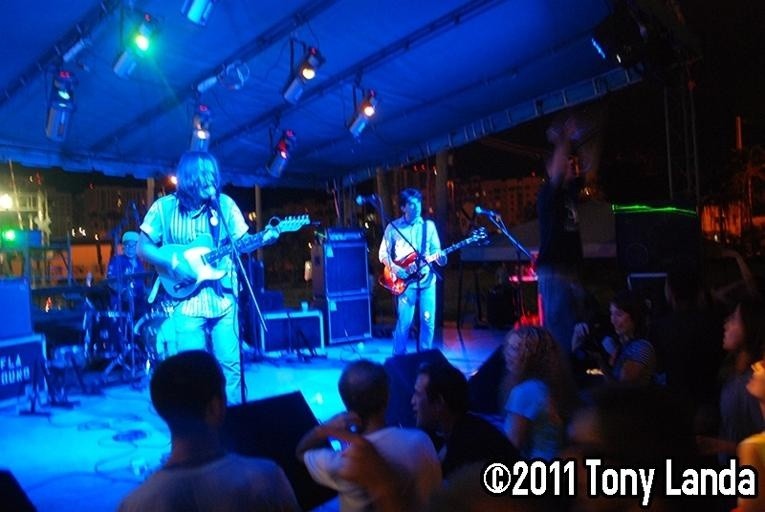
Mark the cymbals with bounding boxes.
[102,271,153,282]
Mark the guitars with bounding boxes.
[378,226,490,296]
[156,215,310,299]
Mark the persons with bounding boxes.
[135,150,279,407]
[533,141,587,331]
[108,231,141,319]
[378,188,448,356]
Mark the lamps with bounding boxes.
[177,0,217,26]
[264,119,304,179]
[279,34,325,111]
[344,73,381,139]
[186,99,220,154]
[111,8,162,78]
[40,56,89,146]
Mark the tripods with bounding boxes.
[105,295,148,389]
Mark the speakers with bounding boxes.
[310,242,369,295]
[217,392,315,458]
[321,295,372,342]
[385,348,446,423]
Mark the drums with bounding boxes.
[141,300,183,360]
[89,311,132,361]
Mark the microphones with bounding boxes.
[475,206,493,216]
[356,195,378,205]
[206,186,218,206]
